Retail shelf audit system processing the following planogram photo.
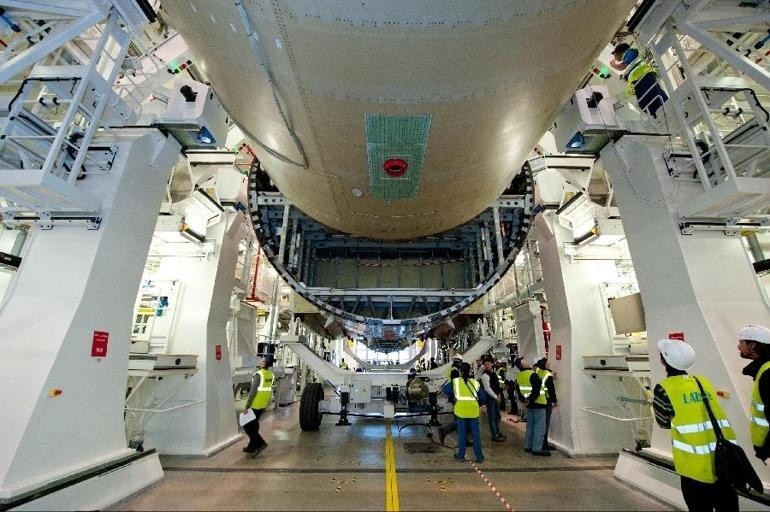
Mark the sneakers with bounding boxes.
[525,442,557,456]
[455,439,484,463]
[438,426,446,445]
[243,446,255,453]
[252,442,267,458]
[491,434,507,442]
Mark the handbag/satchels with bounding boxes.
[712,438,764,496]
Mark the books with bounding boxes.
[239,407,257,427]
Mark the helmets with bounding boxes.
[737,324,770,346]
[453,352,463,361]
[658,338,696,371]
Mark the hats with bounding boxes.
[513,356,524,364]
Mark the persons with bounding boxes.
[438,352,474,448]
[735,322,770,467]
[609,43,668,120]
[338,357,348,370]
[449,362,486,463]
[242,353,276,459]
[651,337,742,511]
[475,356,520,443]
[415,357,438,374]
[514,355,553,457]
[531,356,559,450]
